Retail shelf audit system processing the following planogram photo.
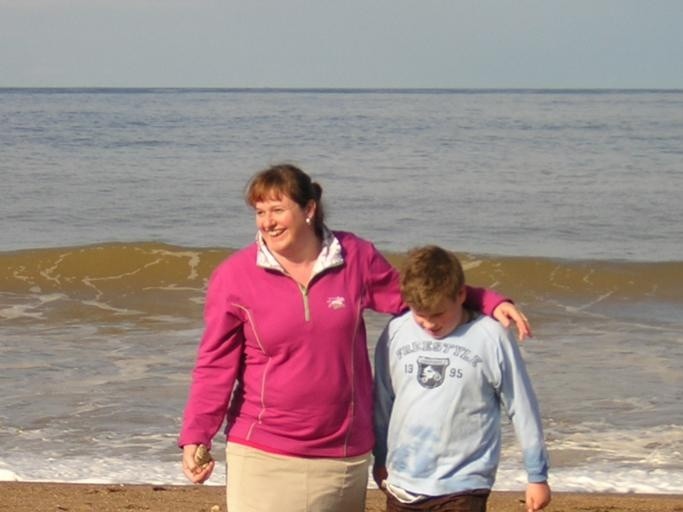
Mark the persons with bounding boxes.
[370,244,552,512]
[177,164,533,512]
